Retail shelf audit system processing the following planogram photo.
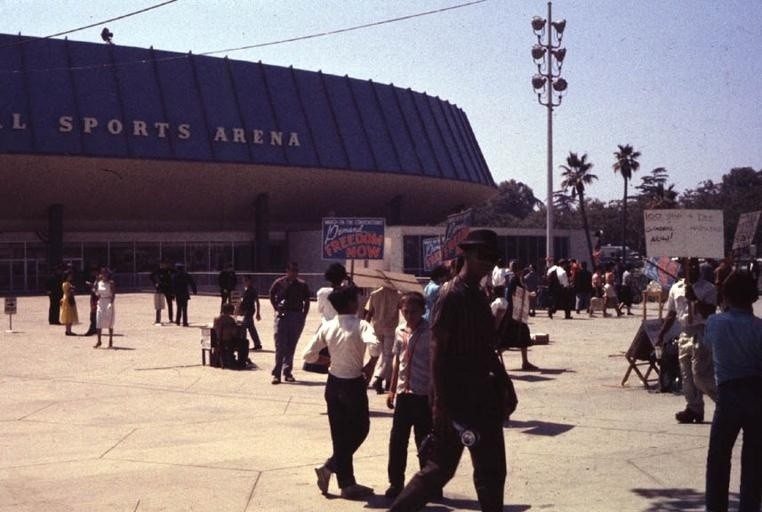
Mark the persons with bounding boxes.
[240,273,263,352]
[45,265,64,324]
[214,303,250,368]
[218,263,237,313]
[150,258,174,326]
[94,265,116,348]
[84,264,98,336]
[268,260,310,384]
[171,262,197,326]
[58,271,79,336]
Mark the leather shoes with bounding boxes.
[50,320,78,336]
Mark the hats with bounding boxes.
[458,228,507,250]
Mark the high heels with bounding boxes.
[93,340,113,348]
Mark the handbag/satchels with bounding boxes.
[449,365,518,427]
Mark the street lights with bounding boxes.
[531,0,568,274]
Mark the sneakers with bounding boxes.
[528,308,635,319]
[340,480,373,499]
[676,408,706,424]
[371,375,385,395]
[154,318,189,327]
[521,362,539,371]
[315,463,330,493]
[383,485,443,499]
[222,344,296,385]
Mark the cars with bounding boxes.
[594,244,760,267]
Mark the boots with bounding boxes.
[83,313,97,337]
